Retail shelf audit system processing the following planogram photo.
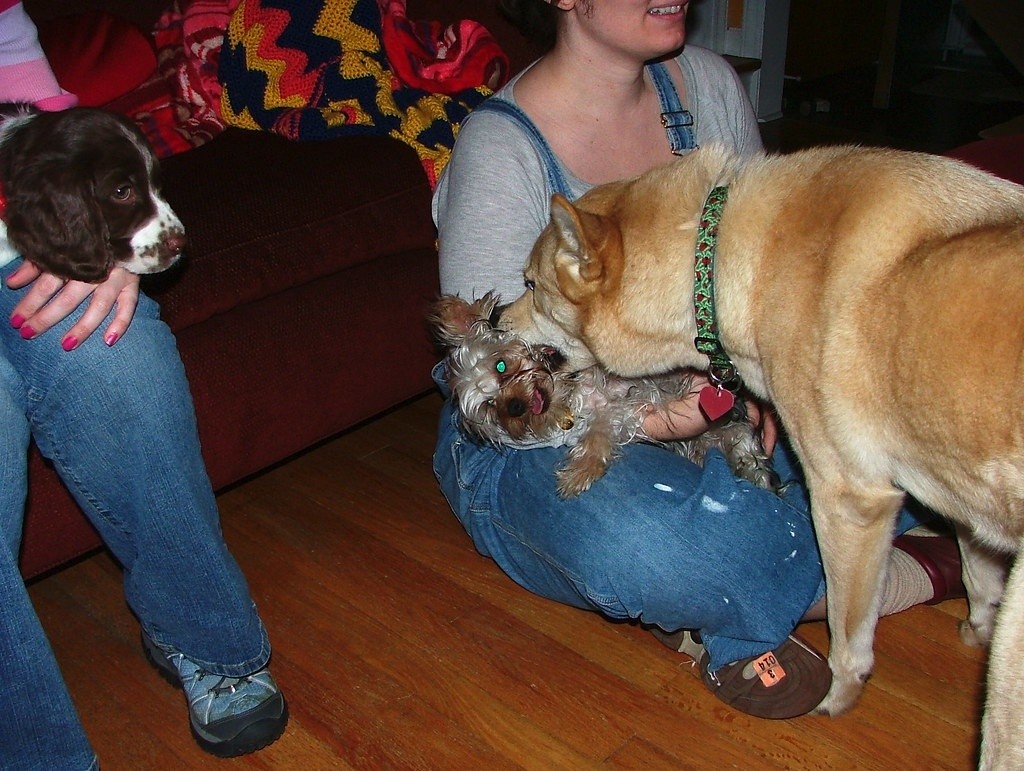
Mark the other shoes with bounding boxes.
[649,624,833,719]
[893,536,969,605]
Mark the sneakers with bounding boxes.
[141,626,289,757]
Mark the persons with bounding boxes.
[431,0,969,719]
[0,1,290,771]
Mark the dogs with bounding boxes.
[0,97,187,285]
[431,145,1024,771]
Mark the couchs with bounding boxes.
[19,0,554,582]
